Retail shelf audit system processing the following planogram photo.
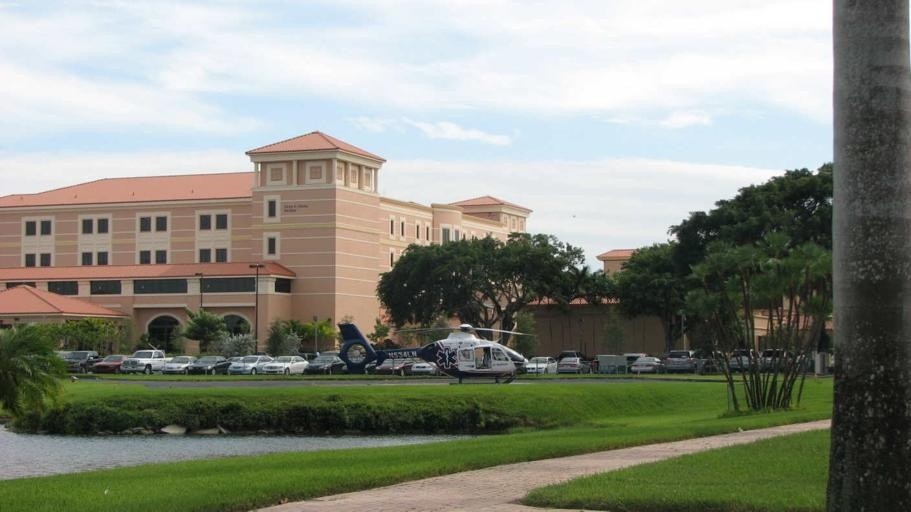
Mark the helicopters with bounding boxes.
[336,319,539,387]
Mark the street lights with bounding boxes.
[195,272,203,311]
[249,264,266,354]
[312,314,320,353]
